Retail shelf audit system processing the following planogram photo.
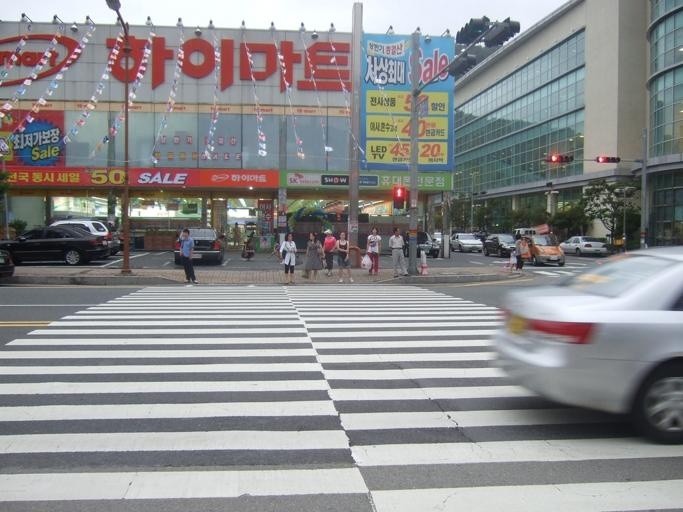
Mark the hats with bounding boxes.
[324,229,332,235]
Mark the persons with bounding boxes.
[366,226,382,276]
[232,222,241,248]
[178,229,199,284]
[267,241,281,262]
[279,233,297,284]
[388,227,408,278]
[515,233,524,272]
[303,229,355,283]
[510,248,517,273]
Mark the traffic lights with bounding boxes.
[597,157,620,163]
[485,21,520,47]
[448,54,476,77]
[393,186,405,209]
[550,155,574,162]
[456,16,490,43]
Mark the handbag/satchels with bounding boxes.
[317,247,323,255]
[520,246,529,254]
[302,270,308,278]
[282,250,287,259]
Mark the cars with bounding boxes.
[516,235,565,266]
[0,220,121,279]
[496,246,683,445]
[483,234,516,258]
[174,228,224,265]
[403,231,441,258]
[449,233,483,253]
[559,236,609,257]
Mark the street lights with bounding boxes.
[107,0,129,268]
[614,187,636,239]
[544,191,559,214]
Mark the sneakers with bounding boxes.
[328,272,354,283]
[183,278,198,284]
